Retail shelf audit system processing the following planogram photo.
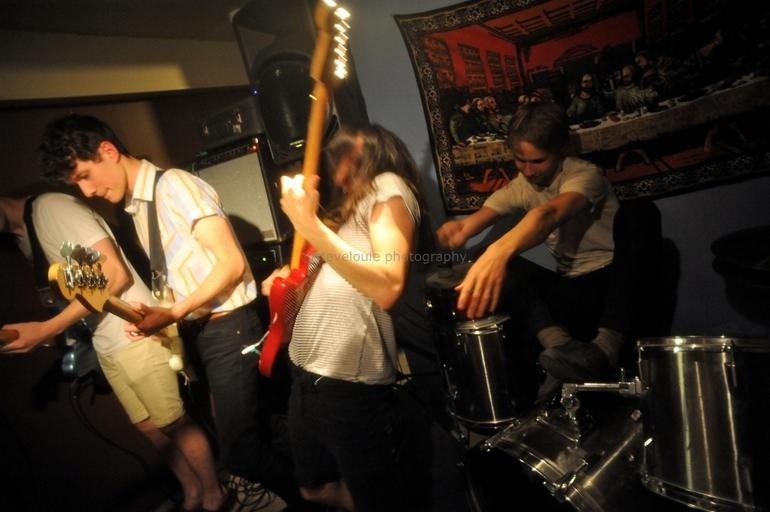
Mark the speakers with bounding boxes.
[176,132,292,246]
[227,0,372,167]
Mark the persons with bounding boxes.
[434,98,669,383]
[0,191,232,511]
[446,52,666,146]
[258,122,443,509]
[32,112,267,486]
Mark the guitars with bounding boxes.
[258,0,351,380]
[47,243,193,382]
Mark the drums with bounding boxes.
[471,385,640,512]
[439,314,519,428]
[635,336,766,510]
